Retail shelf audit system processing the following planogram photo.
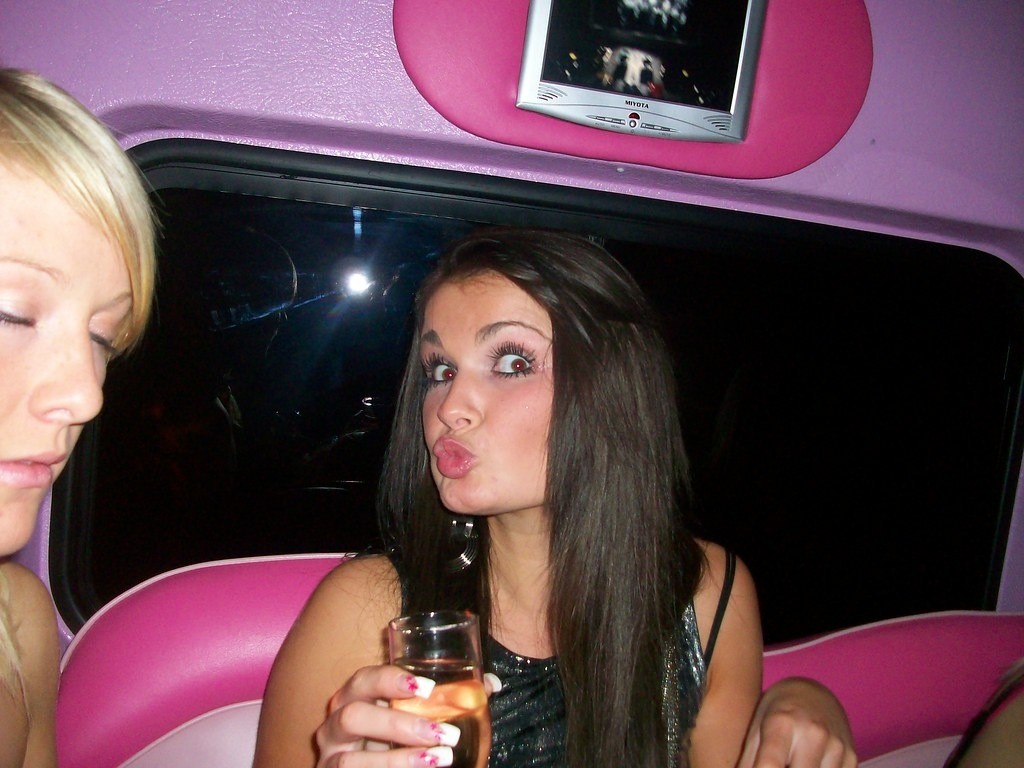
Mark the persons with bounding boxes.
[0,68,156,768]
[252,227,858,768]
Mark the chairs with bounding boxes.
[54,551,361,768]
[762,609,1022,768]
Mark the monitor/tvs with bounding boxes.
[516,0,768,142]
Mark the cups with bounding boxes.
[388,612,492,768]
